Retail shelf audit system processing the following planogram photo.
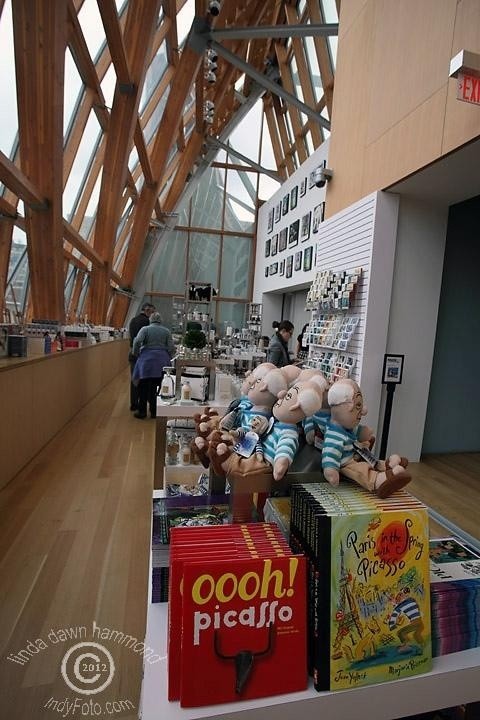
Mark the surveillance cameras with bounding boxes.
[315,174,326,188]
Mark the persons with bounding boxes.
[128,302,156,411]
[130,312,177,420]
[266,319,300,369]
[182,323,206,348]
[293,322,310,361]
[188,360,412,499]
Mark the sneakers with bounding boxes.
[134,412,145,419]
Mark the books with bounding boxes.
[7,334,82,358]
[166,479,480,711]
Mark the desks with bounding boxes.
[154,385,236,490]
[209,462,320,523]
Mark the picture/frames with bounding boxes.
[265,160,325,278]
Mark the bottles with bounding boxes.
[180,381,191,401]
[182,448,191,466]
[167,430,179,464]
[160,374,173,396]
[45,335,51,354]
[168,372,174,396]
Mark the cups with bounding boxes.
[188,314,208,321]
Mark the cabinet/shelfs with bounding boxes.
[1,339,134,489]
